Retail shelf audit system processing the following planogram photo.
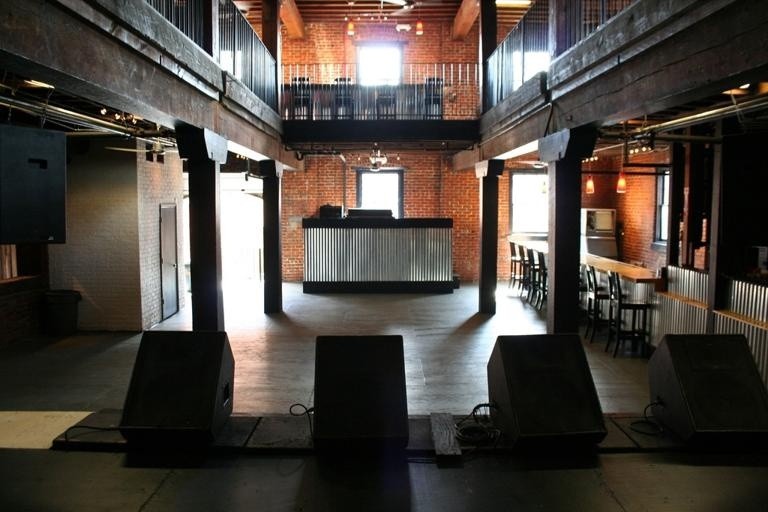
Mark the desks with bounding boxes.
[283,81,444,110]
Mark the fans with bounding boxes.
[104,138,178,156]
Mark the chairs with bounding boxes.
[331,78,354,120]
[290,77,314,122]
[507,240,652,357]
[376,82,397,120]
[424,77,444,121]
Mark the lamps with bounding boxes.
[345,0,356,36]
[368,141,387,172]
[615,135,626,194]
[585,154,595,195]
[415,0,424,35]
